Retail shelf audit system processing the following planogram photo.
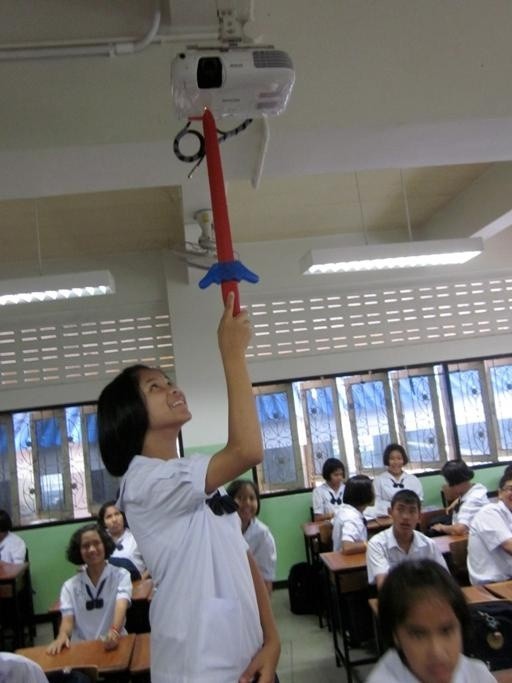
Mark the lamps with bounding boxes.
[0,197,118,306]
[301,165,485,276]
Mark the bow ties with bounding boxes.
[455,503,459,512]
[206,495,239,515]
[331,498,341,504]
[115,544,123,550]
[393,484,403,488]
[86,598,104,610]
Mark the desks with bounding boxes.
[0,559,156,683]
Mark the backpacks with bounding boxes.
[288,562,337,615]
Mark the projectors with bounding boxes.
[169,43,297,120]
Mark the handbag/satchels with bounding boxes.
[464,603,511,670]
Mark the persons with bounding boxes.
[0,509,26,563]
[45,525,134,683]
[361,557,497,683]
[98,500,150,579]
[308,440,512,593]
[93,289,282,683]
[0,650,52,683]
[227,479,277,601]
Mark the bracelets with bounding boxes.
[109,625,119,633]
